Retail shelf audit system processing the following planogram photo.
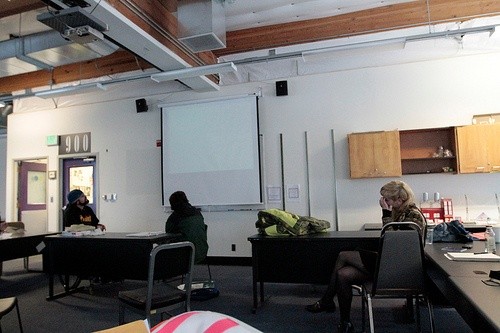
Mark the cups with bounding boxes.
[425,229,433,244]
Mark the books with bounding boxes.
[444,253,500,262]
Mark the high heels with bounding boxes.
[338,321,355,333]
[304,299,336,313]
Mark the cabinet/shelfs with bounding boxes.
[348,123,500,179]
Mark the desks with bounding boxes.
[0,232,191,301]
[248,224,500,333]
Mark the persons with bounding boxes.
[164,190,209,262]
[64,189,106,232]
[304,181,427,333]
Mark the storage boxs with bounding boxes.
[472,113,500,125]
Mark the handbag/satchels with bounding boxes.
[428,220,473,243]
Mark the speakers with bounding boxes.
[136,99,147,112]
[276,80,289,95]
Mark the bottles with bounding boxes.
[484,225,497,254]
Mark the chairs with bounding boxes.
[0,297,23,333]
[117,242,195,326]
[181,224,212,284]
[360,221,436,333]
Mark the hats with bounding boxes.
[67,189,84,205]
[169,191,188,210]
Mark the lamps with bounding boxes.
[151,62,237,83]
[36,83,106,98]
